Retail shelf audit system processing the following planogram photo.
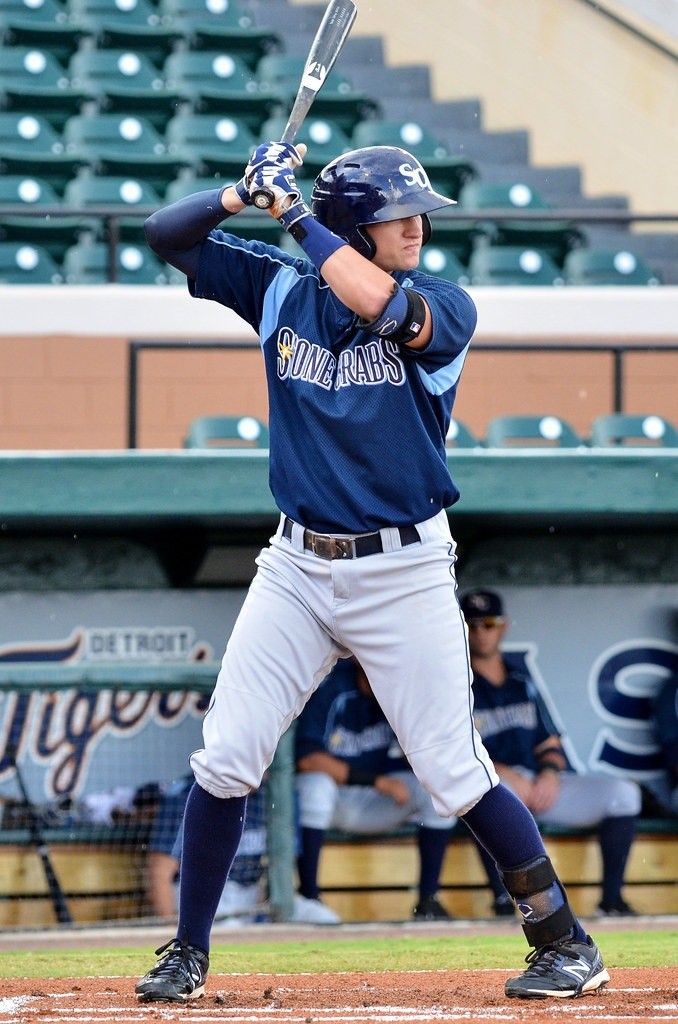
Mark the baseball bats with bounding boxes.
[5,743,74,922]
[250,0,358,210]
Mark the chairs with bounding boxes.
[181,414,678,450]
[0,0,678,292]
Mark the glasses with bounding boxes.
[468,618,504,632]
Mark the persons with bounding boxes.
[459,587,643,917]
[132,142,610,1002]
[143,760,344,926]
[291,656,459,922]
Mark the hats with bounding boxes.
[457,589,503,619]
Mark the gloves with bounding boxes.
[249,165,313,232]
[235,141,306,206]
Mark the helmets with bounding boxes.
[309,145,460,264]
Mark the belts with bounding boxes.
[280,518,422,561]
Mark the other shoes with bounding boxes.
[488,900,517,918]
[414,903,454,921]
[594,895,637,915]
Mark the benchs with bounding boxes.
[0,800,678,921]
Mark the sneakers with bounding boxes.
[133,938,210,1002]
[504,935,612,999]
